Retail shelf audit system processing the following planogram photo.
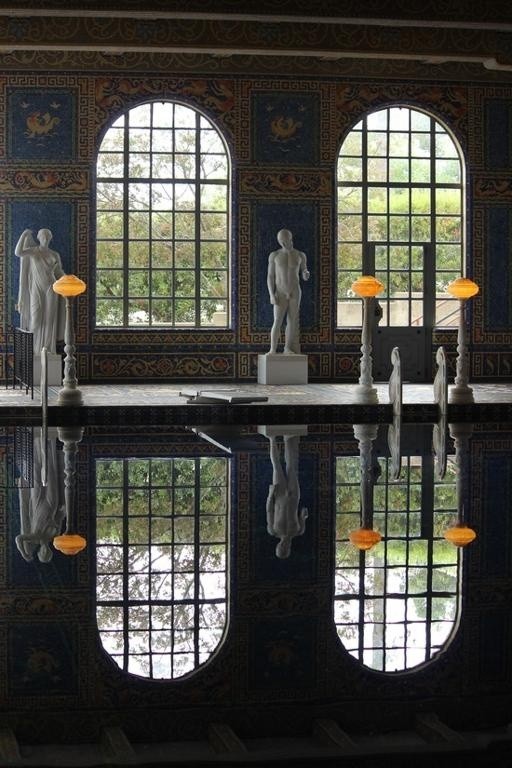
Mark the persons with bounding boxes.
[10,426,67,562]
[265,228,309,355]
[264,435,312,562]
[13,225,69,354]
